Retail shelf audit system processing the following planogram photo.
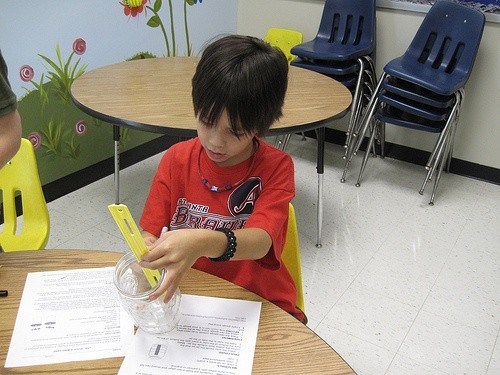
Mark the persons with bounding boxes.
[134,35,307,322]
[0,48,23,169]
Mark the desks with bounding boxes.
[0,249,357,375]
[71,57,353,247]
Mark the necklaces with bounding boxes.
[197,144,258,193]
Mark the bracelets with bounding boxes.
[208,227,236,262]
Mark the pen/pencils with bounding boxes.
[161,226,168,236]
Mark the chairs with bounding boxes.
[266,0,485,206]
[0,137,50,250]
[280,202,303,311]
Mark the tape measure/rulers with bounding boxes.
[108,204,161,288]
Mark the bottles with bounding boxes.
[112,251,182,335]
